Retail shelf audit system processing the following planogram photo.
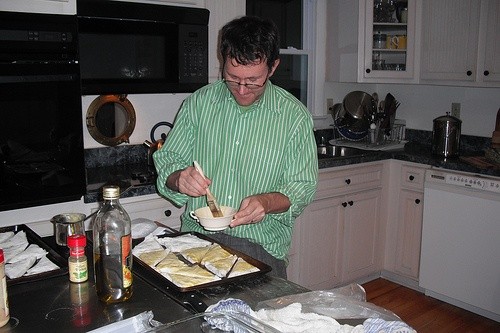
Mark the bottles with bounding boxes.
[93,186,134,305]
[431,112,462,160]
[67,235,88,283]
[0,249,10,329]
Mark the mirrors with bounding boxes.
[88,95,136,147]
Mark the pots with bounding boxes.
[50,186,133,247]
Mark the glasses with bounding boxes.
[221,61,271,90]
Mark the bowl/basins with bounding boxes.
[190,206,238,232]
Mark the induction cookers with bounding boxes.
[86,163,158,203]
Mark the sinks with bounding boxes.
[316,143,366,162]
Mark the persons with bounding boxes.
[152,14,319,279]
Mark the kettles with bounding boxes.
[144,121,173,154]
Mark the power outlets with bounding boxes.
[327,98,334,113]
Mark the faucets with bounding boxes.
[313,127,328,147]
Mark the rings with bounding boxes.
[251,219,254,223]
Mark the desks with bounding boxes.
[0,221,371,333]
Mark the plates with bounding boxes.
[86,95,136,146]
[333,91,376,141]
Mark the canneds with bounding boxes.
[0,249,10,328]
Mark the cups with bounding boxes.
[386,35,406,49]
[401,8,407,23]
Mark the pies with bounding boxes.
[128,233,260,288]
[0,229,60,278]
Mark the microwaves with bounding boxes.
[76,1,210,96]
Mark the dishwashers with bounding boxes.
[418,168,500,323]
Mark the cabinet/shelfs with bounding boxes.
[301,164,384,289]
[392,164,427,278]
[359,0,415,83]
[91,196,187,239]
[419,0,500,82]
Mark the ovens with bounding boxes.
[0,10,85,212]
